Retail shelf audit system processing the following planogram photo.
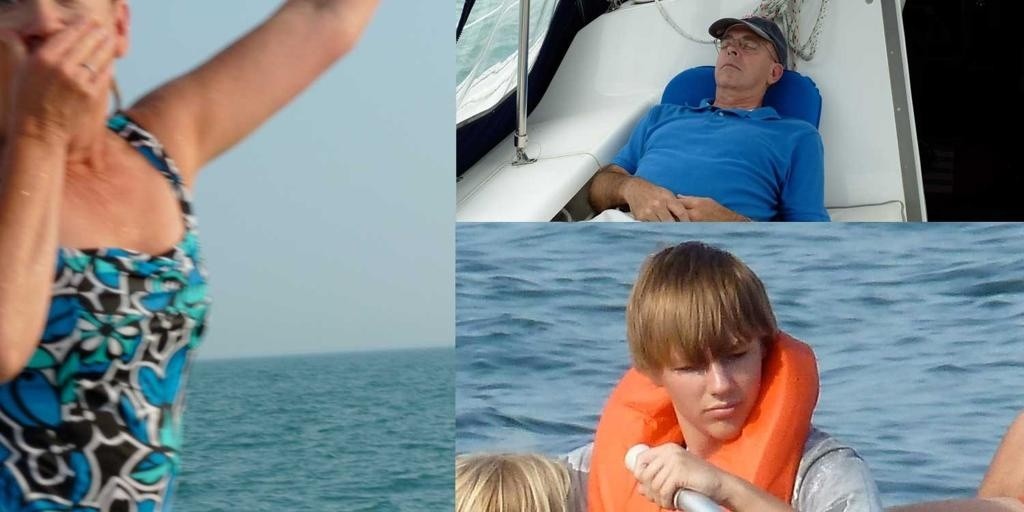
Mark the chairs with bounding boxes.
[662,66,822,220]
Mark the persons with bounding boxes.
[585,15,833,220]
[455,449,575,512]
[3,3,392,512]
[564,235,883,511]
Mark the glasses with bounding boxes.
[713,36,777,62]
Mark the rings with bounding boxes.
[80,63,97,81]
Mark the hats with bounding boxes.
[708,16,788,65]
